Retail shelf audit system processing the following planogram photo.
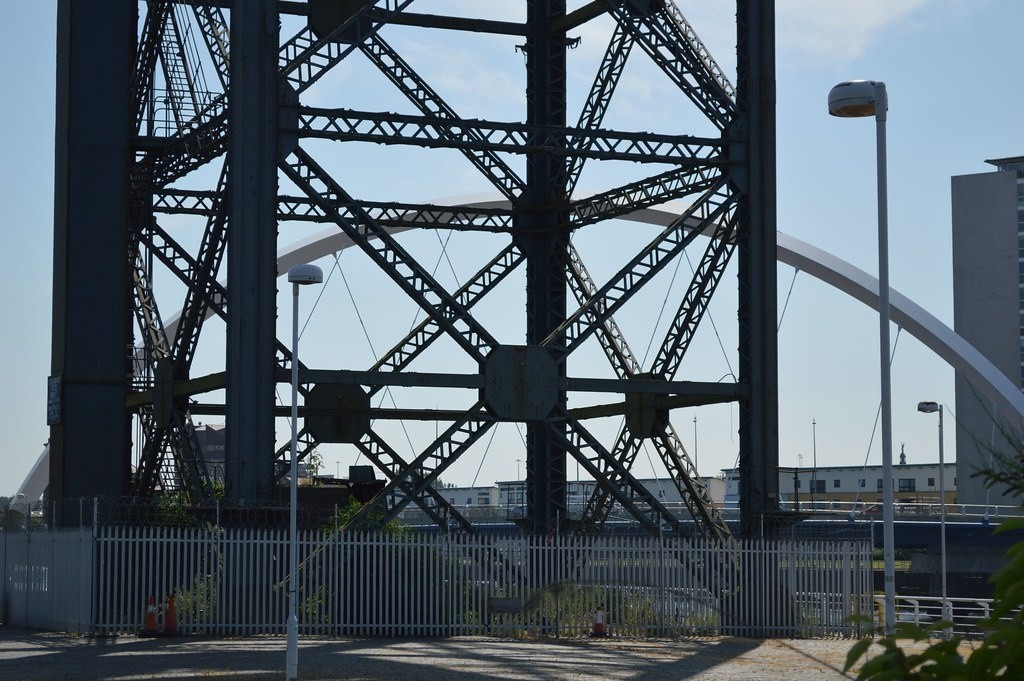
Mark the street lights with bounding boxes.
[827,79,899,650]
[515,459,522,481]
[919,400,949,641]
[812,418,818,513]
[693,415,698,470]
[284,262,327,681]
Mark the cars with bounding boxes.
[860,504,884,517]
[512,506,527,517]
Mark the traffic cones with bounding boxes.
[160,597,180,638]
[139,595,161,638]
